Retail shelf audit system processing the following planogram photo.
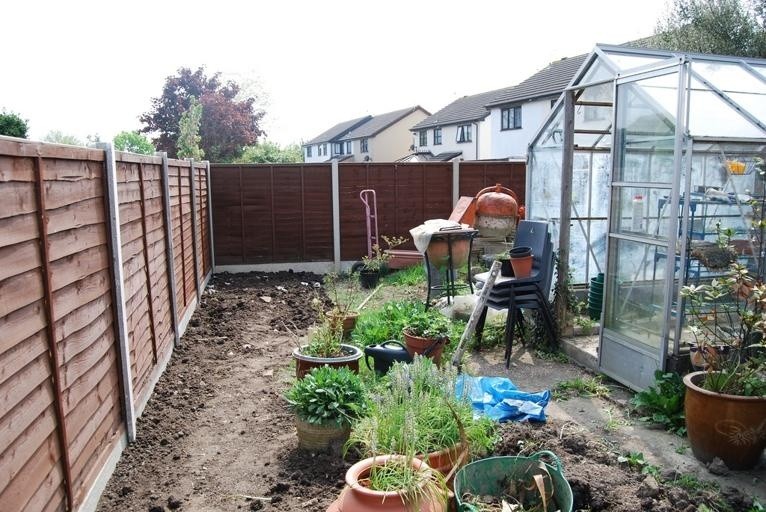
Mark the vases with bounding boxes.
[416,216,481,322]
[499,258,515,279]
[452,447,573,511]
[508,245,535,279]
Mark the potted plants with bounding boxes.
[359,255,392,289]
[344,350,501,495]
[286,364,366,451]
[682,225,766,469]
[401,318,453,369]
[325,389,457,512]
[319,268,363,336]
[285,283,365,386]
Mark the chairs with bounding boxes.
[470,217,569,370]
